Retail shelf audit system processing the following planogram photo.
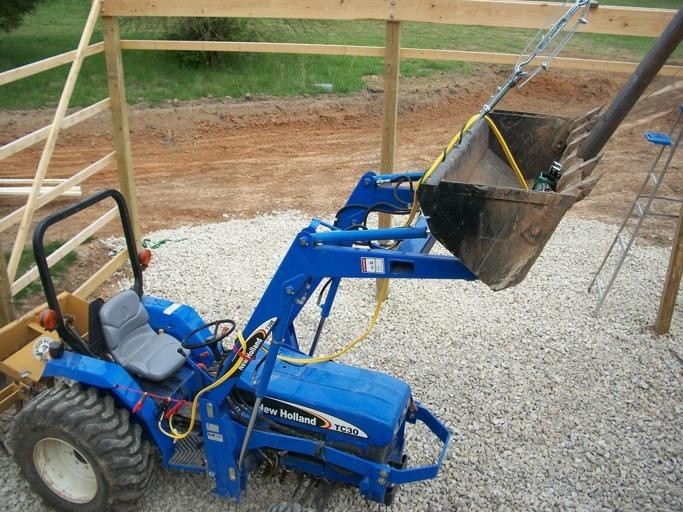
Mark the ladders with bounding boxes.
[587,104,683,312]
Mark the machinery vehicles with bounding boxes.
[10,98,613,511]
